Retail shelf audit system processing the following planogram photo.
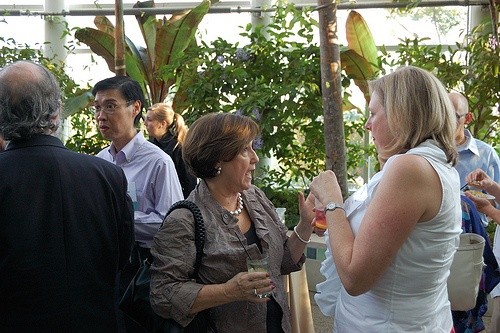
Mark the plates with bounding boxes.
[460,191,495,200]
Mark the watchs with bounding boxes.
[324,202,346,215]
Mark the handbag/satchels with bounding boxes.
[114,200,215,331]
[445,233,485,314]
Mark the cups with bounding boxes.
[247,254,273,298]
[314,196,328,229]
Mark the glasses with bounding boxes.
[455,112,469,120]
[93,99,139,116]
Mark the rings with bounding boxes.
[255,289,258,296]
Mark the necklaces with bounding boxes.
[229,193,244,215]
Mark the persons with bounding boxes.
[447,93,500,333]
[151,112,316,333]
[0,59,133,333]
[464,168,500,333]
[92,76,185,333]
[145,103,197,201]
[310,66,462,333]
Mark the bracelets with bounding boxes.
[294,226,311,243]
